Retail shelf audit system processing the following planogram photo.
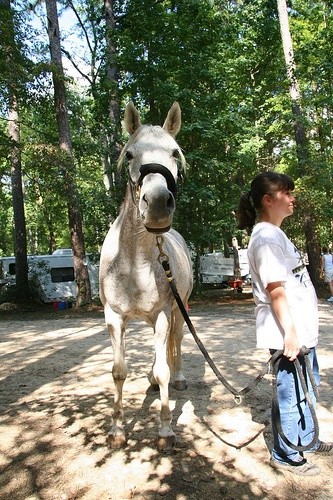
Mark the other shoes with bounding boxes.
[327,297,333,302]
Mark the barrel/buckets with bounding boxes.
[52,300,74,310]
[228,281,242,288]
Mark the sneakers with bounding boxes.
[302,440,333,455]
[269,455,321,476]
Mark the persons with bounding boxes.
[235,172,333,476]
[319,246,333,302]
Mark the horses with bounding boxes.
[94,98,197,454]
